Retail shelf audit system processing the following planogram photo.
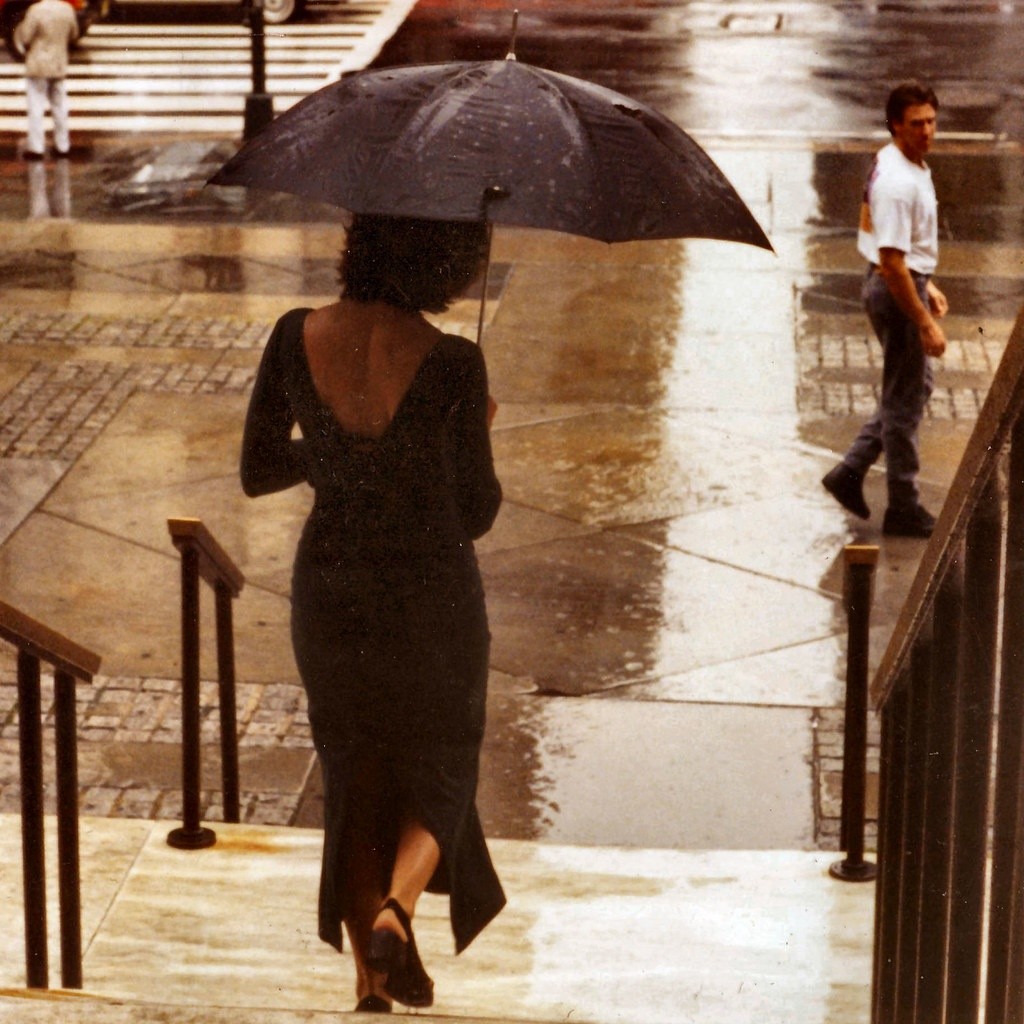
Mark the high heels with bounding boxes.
[355,995,391,1015]
[365,899,434,1008]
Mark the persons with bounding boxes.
[13,0,79,161]
[239,212,505,1012]
[822,82,948,535]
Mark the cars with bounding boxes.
[56,0,346,27]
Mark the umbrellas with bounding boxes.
[201,10,778,347]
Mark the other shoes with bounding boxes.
[822,462,871,520]
[50,146,70,157]
[883,502,937,538]
[23,150,44,161]
